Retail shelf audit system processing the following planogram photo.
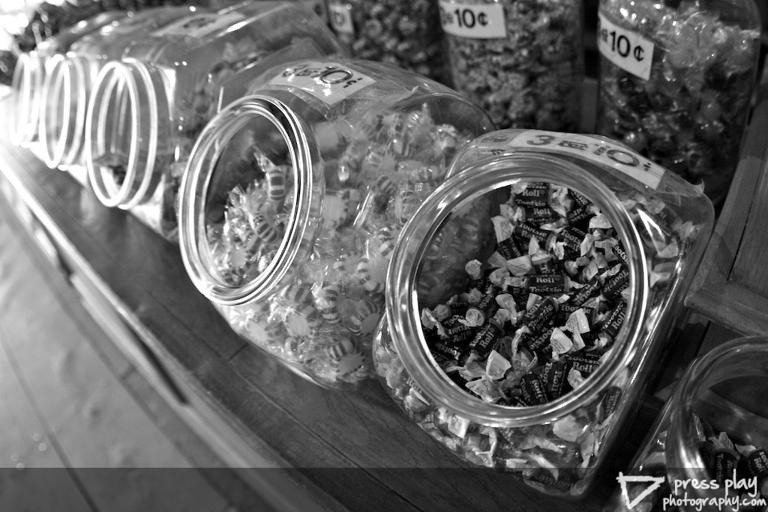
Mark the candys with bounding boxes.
[325,1,439,76]
[594,1,764,210]
[162,73,456,391]
[606,412,768,512]
[371,127,702,500]
[87,19,335,233]
[434,1,590,128]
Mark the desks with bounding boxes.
[676,52,768,382]
[0,138,658,512]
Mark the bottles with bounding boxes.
[178,58,498,391]
[438,1,564,129]
[316,1,437,78]
[11,9,127,161]
[371,127,715,499]
[41,7,209,190]
[85,0,326,247]
[592,0,764,204]
[613,334,767,512]
